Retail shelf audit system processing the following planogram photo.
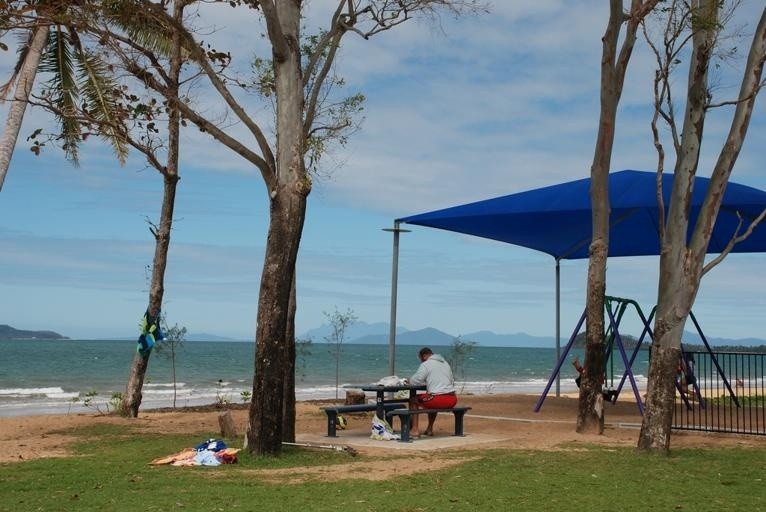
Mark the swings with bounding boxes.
[573,297,627,402]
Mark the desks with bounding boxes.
[361,384,429,435]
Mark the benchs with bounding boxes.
[325,402,405,437]
[386,406,472,441]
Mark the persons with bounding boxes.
[405,348,457,437]
[573,352,695,402]
